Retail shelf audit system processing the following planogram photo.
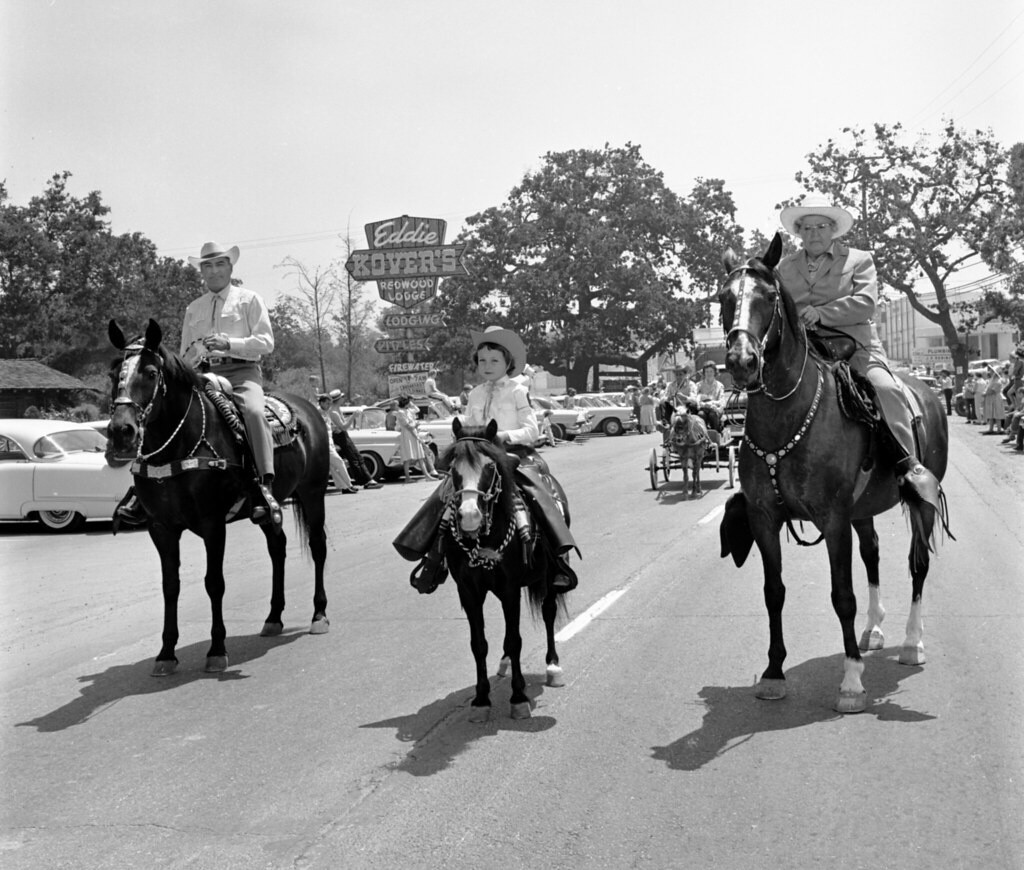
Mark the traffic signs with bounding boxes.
[388,361,440,374]
[374,336,430,354]
[383,313,447,328]
[345,242,470,281]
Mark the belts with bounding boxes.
[199,357,256,367]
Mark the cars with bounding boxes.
[917,376,942,390]
[967,359,1003,378]
[0,418,135,533]
[379,399,467,461]
[526,391,640,448]
[336,405,435,485]
[715,364,753,437]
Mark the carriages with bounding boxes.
[645,399,741,497]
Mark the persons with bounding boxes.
[308,389,383,493]
[417,324,579,595]
[937,341,1024,454]
[567,387,594,426]
[907,363,933,377]
[624,372,667,434]
[422,369,473,412]
[666,360,724,433]
[178,241,276,524]
[769,192,942,514]
[385,394,444,483]
[541,410,558,449]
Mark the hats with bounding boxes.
[187,242,239,272]
[672,365,691,374]
[329,390,344,402]
[471,326,525,378]
[989,364,1003,380]
[780,195,853,240]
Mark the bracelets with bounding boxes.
[225,338,231,351]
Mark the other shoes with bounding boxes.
[341,488,356,493]
[116,498,143,520]
[426,476,435,482]
[364,481,383,489]
[418,565,438,595]
[552,573,569,591]
[966,420,1023,449]
[405,478,415,483]
[252,493,280,518]
[430,470,440,478]
[349,487,359,491]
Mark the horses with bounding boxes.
[662,405,711,500]
[439,417,579,724]
[107,317,330,677]
[722,229,949,713]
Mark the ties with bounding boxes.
[211,300,217,326]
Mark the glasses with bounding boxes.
[802,223,827,231]
